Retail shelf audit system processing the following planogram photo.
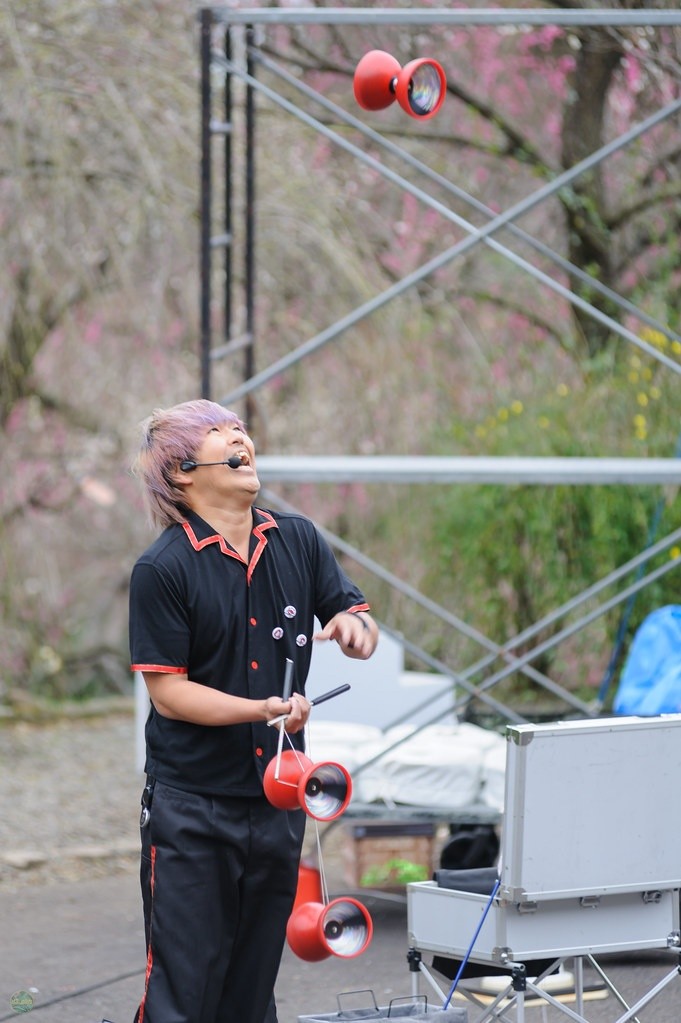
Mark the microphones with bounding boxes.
[179,456,241,471]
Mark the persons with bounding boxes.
[129,398,379,1023]
